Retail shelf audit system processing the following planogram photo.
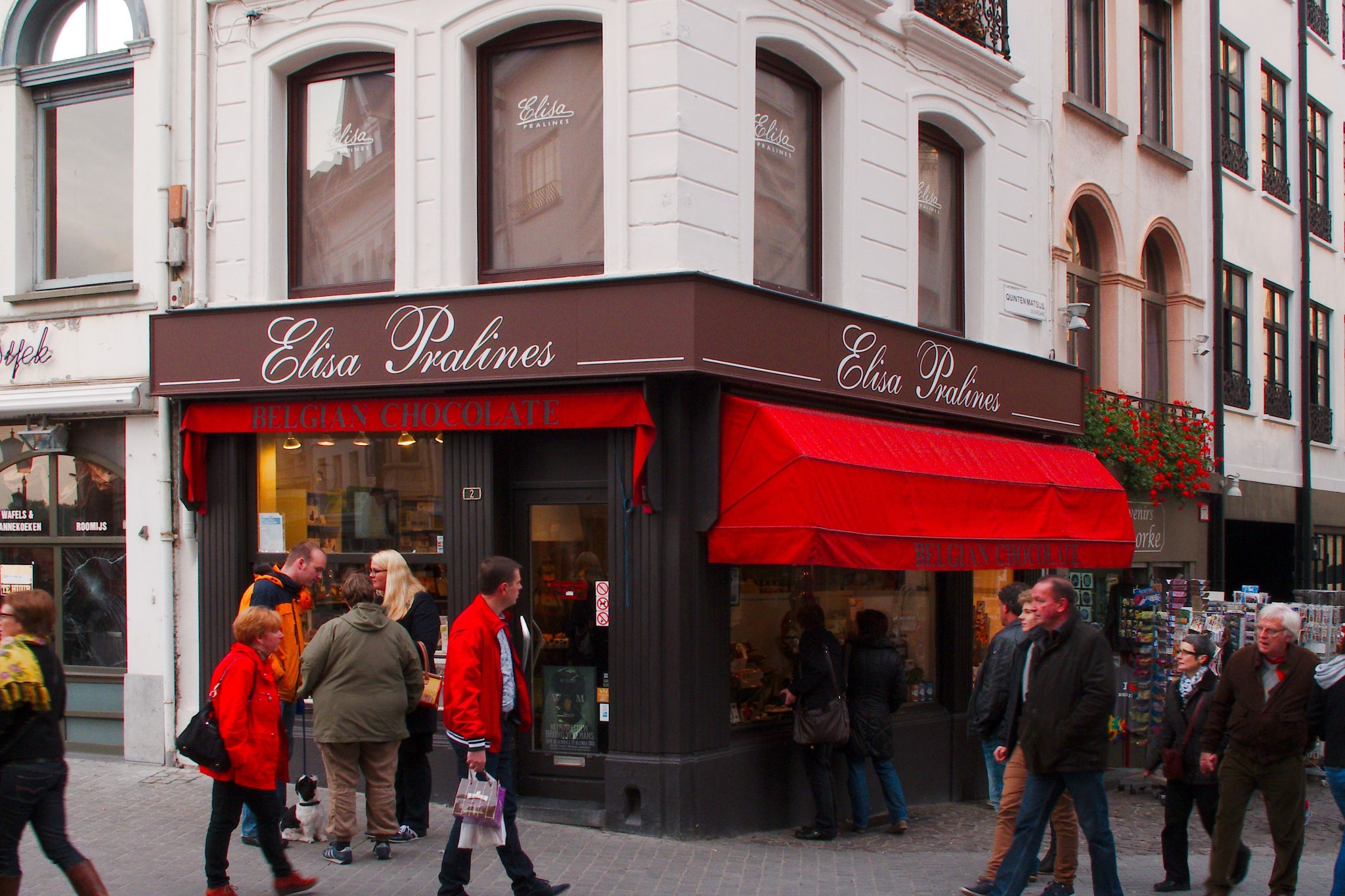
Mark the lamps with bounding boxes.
[278,427,445,450]
[1058,301,1092,334]
[16,412,70,455]
[1219,472,1242,497]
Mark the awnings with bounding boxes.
[704,394,1138,576]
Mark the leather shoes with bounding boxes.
[1229,845,1251,885]
[1153,879,1191,891]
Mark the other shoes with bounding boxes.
[513,879,570,896]
[795,826,834,840]
[1038,844,1057,873]
[1040,880,1075,896]
[437,889,469,896]
[206,885,239,896]
[887,820,907,834]
[274,872,317,896]
[1338,822,1345,829]
[959,882,993,896]
[843,816,865,832]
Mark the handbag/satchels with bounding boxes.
[175,695,232,771]
[453,766,502,826]
[1163,748,1184,778]
[794,694,849,743]
[458,766,506,848]
[418,640,443,711]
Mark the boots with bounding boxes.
[0,871,23,896]
[64,859,110,896]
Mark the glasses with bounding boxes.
[369,569,386,576]
[1254,625,1287,635]
[1175,650,1198,656]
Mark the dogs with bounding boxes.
[280,774,328,844]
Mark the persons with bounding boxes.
[735,642,757,670]
[961,578,1127,895]
[1303,731,1328,827]
[297,571,424,865]
[1313,635,1345,896]
[364,549,442,843]
[1199,602,1322,896]
[563,551,608,680]
[1143,633,1251,891]
[241,541,327,850]
[842,607,912,835]
[0,588,108,896]
[777,597,845,839]
[198,604,320,896]
[435,554,572,895]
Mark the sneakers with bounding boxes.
[241,835,290,846]
[322,846,352,864]
[365,831,375,838]
[389,828,427,842]
[373,844,391,860]
[1304,807,1313,825]
